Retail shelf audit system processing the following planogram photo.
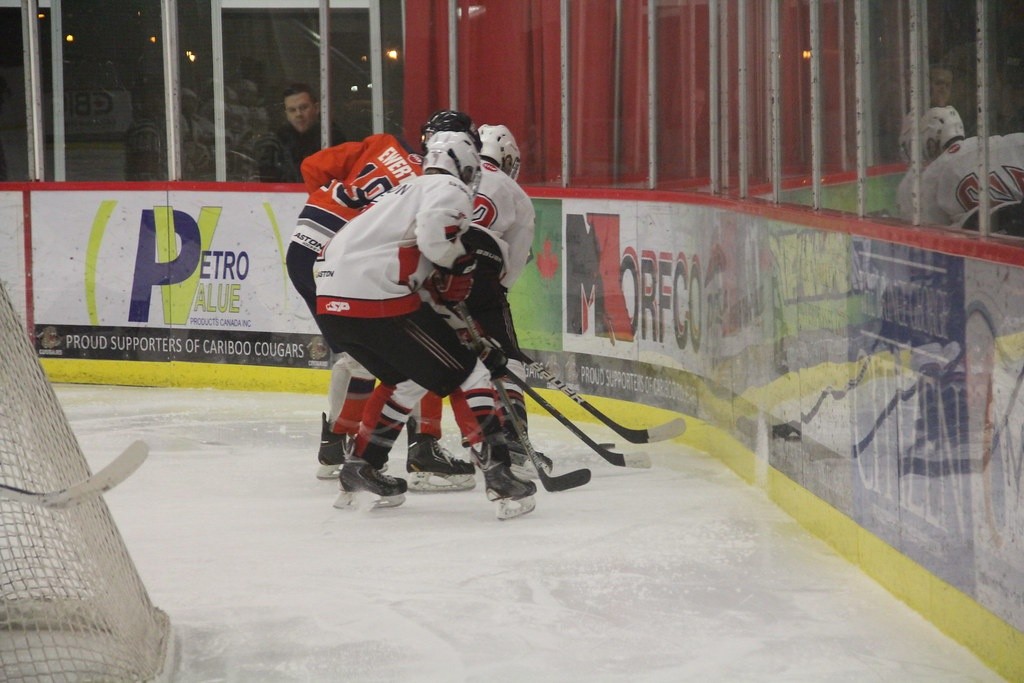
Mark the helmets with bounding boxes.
[919,106,965,162]
[420,110,483,153]
[423,131,482,185]
[898,111,914,167]
[477,124,520,170]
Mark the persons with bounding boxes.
[896,106,1024,238]
[258,85,345,184]
[286,109,553,520]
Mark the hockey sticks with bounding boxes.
[806,383,920,465]
[517,351,687,443]
[459,299,592,492]
[0,440,151,509]
[735,327,886,441]
[503,365,653,470]
[902,369,1024,476]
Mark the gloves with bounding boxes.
[476,335,508,377]
[425,254,478,305]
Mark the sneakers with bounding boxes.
[501,427,553,479]
[471,440,537,520]
[406,417,476,491]
[317,412,388,478]
[333,438,407,509]
